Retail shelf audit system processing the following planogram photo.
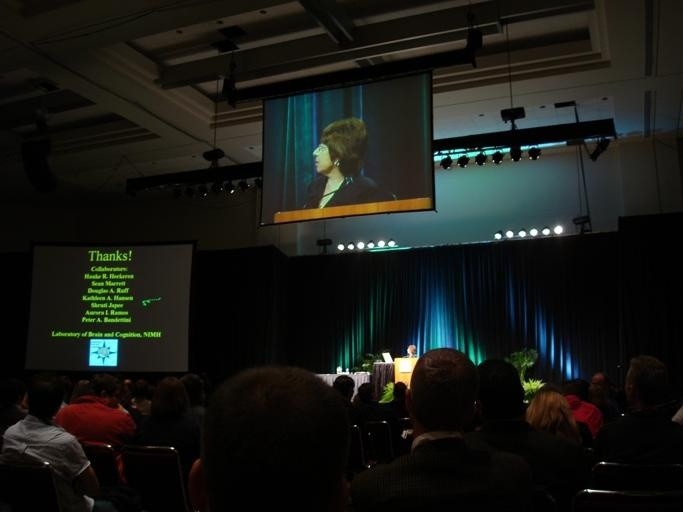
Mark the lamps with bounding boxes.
[172,176,263,202]
[572,213,592,226]
[315,238,333,248]
[200,148,227,163]
[585,141,610,162]
[441,145,542,173]
[336,237,396,252]
[494,224,564,242]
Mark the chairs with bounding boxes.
[569,459,682,511]
[0,439,190,512]
[348,416,414,470]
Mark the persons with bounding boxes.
[301,117,396,209]
[1,346,682,511]
